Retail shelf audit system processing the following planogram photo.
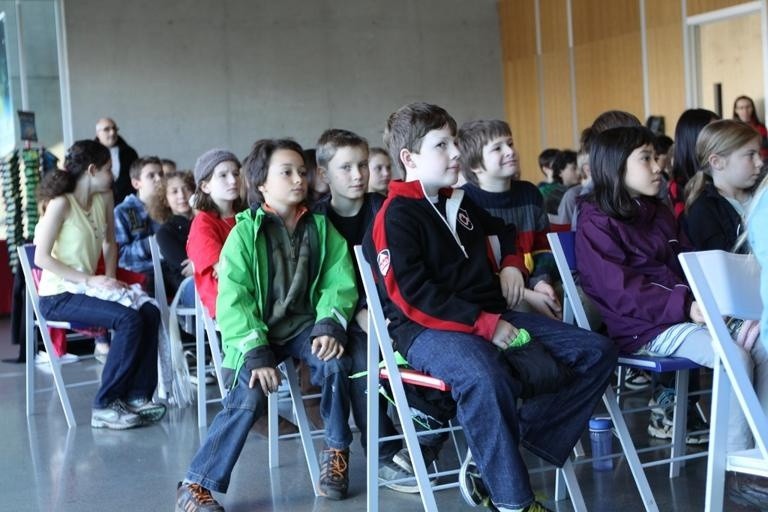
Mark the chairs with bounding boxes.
[111,231,766,511]
[17,243,158,430]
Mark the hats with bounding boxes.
[193,149,243,194]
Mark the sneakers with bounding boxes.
[122,395,168,426]
[91,397,142,431]
[458,444,491,508]
[93,342,111,363]
[482,495,556,512]
[173,479,225,512]
[249,409,299,441]
[377,459,437,494]
[392,443,442,474]
[647,384,680,427]
[726,476,768,512]
[33,348,82,366]
[613,362,651,392]
[317,438,350,500]
[647,405,711,445]
[187,368,217,386]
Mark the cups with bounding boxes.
[586,415,616,470]
